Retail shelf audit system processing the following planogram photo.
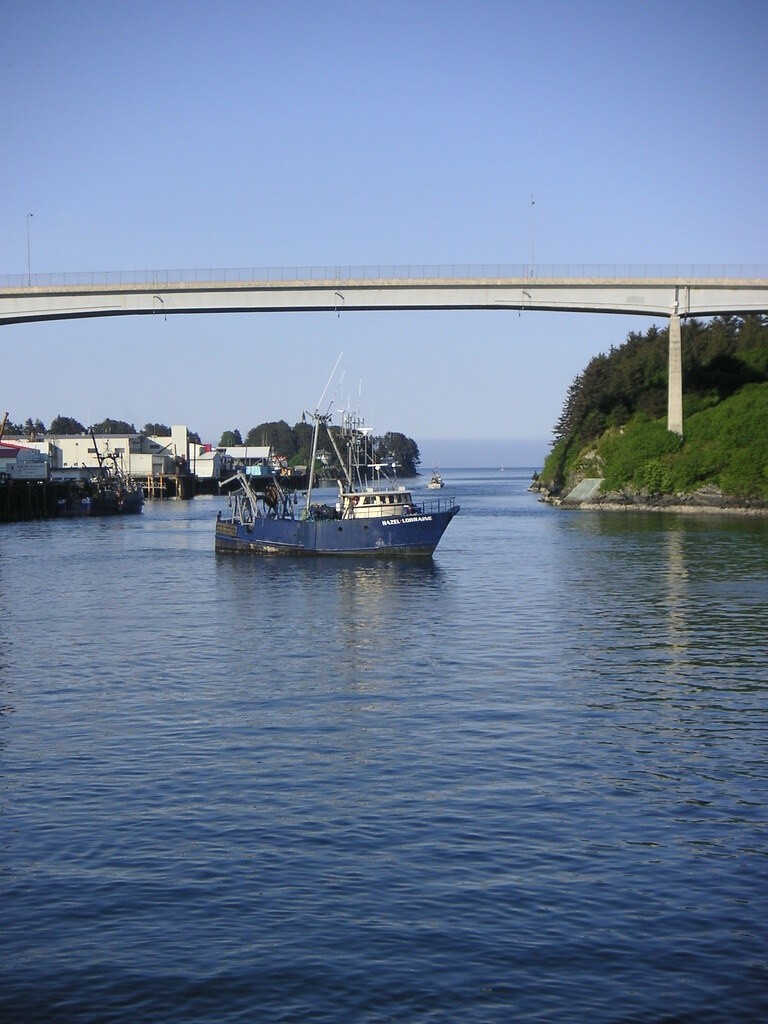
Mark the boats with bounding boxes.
[81,433,145,516]
[212,356,464,564]
[426,472,451,491]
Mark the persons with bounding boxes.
[403,501,417,515]
